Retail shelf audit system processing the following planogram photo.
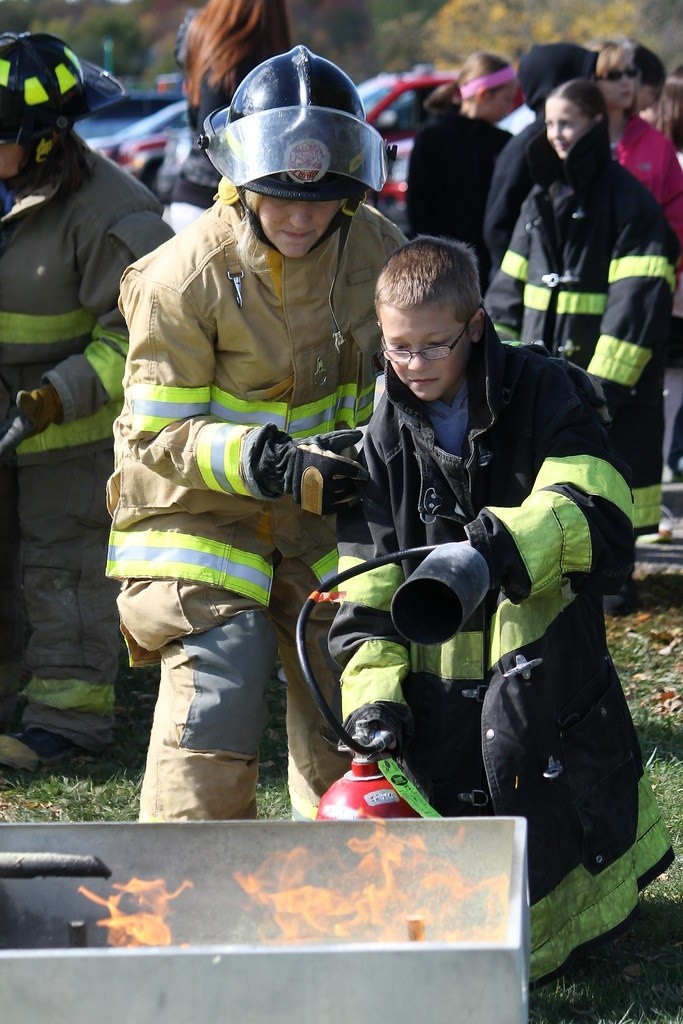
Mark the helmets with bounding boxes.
[197,44,398,202]
[0,31,124,141]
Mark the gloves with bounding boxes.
[17,384,61,439]
[252,424,382,515]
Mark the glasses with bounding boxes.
[596,65,638,82]
[380,299,484,360]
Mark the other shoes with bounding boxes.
[637,526,673,543]
[0,726,110,772]
[603,575,636,615]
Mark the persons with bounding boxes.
[0,33,174,775]
[103,0,683,992]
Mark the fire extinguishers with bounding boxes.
[296,539,488,820]
